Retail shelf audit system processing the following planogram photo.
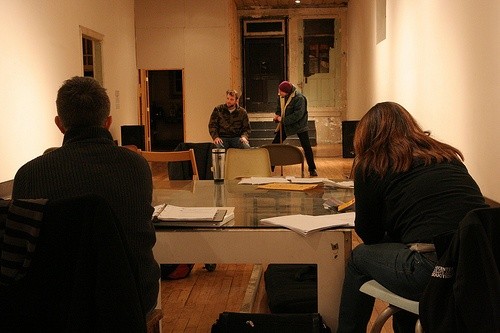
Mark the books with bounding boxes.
[151,203,234,227]
[259,212,356,237]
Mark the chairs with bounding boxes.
[360,206,500,333]
[0,193,145,333]
[44,144,305,181]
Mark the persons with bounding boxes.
[335,102,492,333]
[206,89,252,180]
[271,81,318,176]
[11,76,161,333]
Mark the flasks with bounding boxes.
[211,148,226,181]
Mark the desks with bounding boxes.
[167,143,219,180]
[151,179,356,333]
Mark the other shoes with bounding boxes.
[310,170,317,176]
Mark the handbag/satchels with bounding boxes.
[211,312,331,333]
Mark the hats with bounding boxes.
[278,81,292,93]
